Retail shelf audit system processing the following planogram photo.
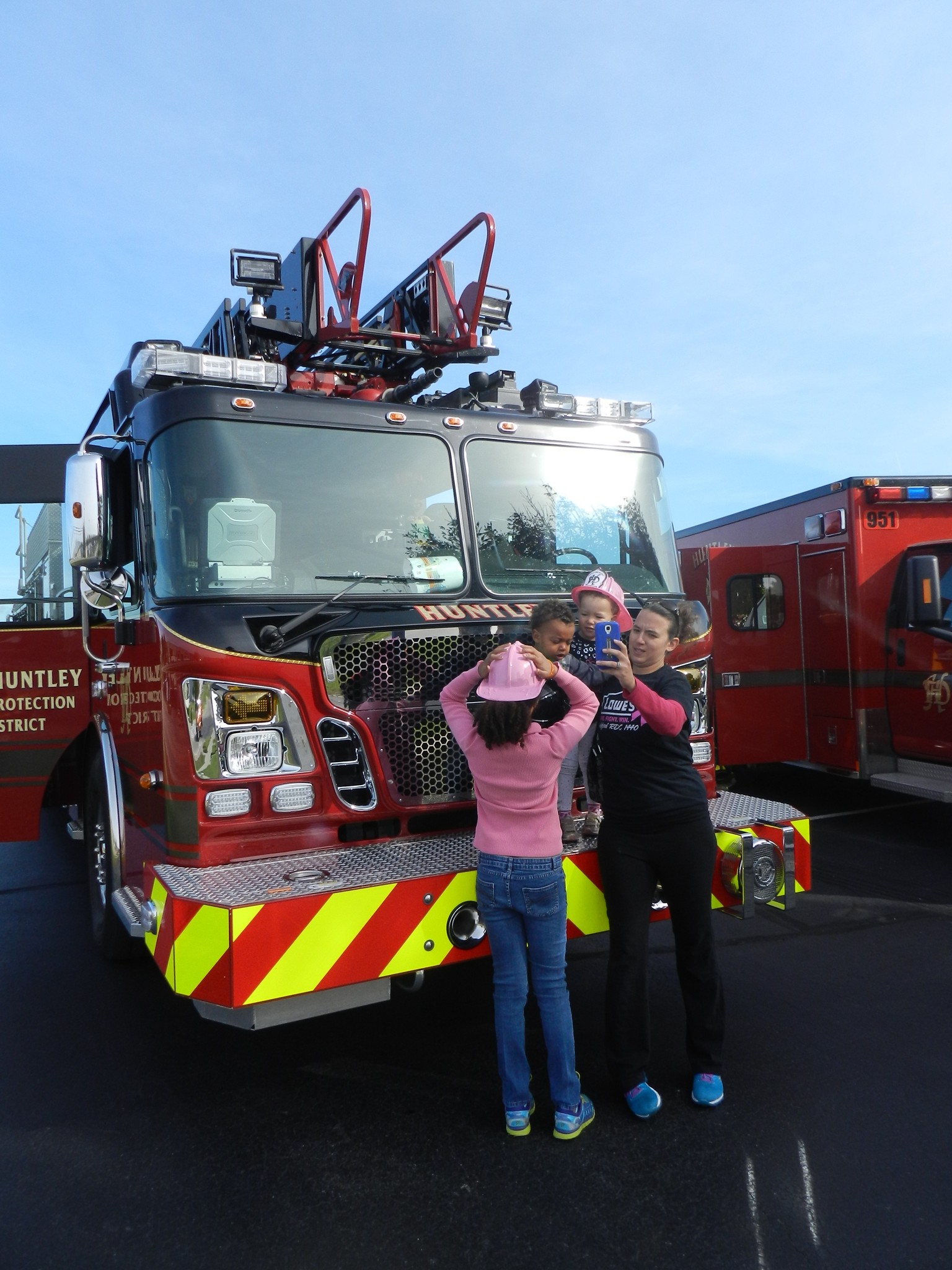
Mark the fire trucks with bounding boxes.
[1,186,812,1031]
[674,477,952,806]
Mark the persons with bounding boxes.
[529,569,726,1119]
[440,640,600,1140]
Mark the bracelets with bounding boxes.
[546,658,554,679]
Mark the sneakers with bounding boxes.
[560,815,577,842]
[553,1071,596,1139]
[582,811,600,835]
[506,1073,535,1137]
[623,1071,661,1119]
[691,1071,723,1106]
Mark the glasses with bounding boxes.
[645,596,679,639]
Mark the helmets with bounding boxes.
[475,640,546,701]
[571,565,634,633]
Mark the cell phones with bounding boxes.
[594,621,622,669]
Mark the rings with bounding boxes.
[616,661,620,669]
